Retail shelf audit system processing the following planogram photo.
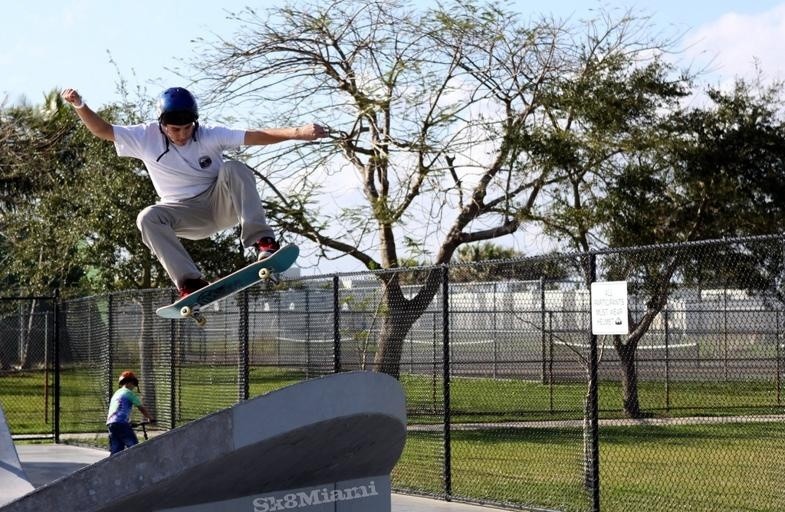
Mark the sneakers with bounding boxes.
[179,280,201,297]
[258,241,276,261]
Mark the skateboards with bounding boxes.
[155,242,299,328]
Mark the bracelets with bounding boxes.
[70,101,85,109]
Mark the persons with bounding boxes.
[106,370,154,455]
[59,86,332,303]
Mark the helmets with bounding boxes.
[158,88,200,126]
[118,371,135,387]
